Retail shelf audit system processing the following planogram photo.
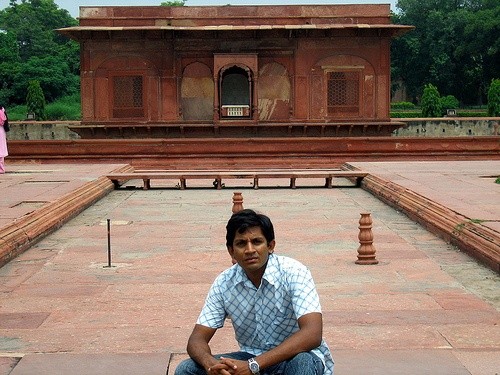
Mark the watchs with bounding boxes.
[248,358,259,374]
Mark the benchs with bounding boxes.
[107,170,369,190]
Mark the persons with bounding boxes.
[174,209,335,374]
[0,105,10,174]
